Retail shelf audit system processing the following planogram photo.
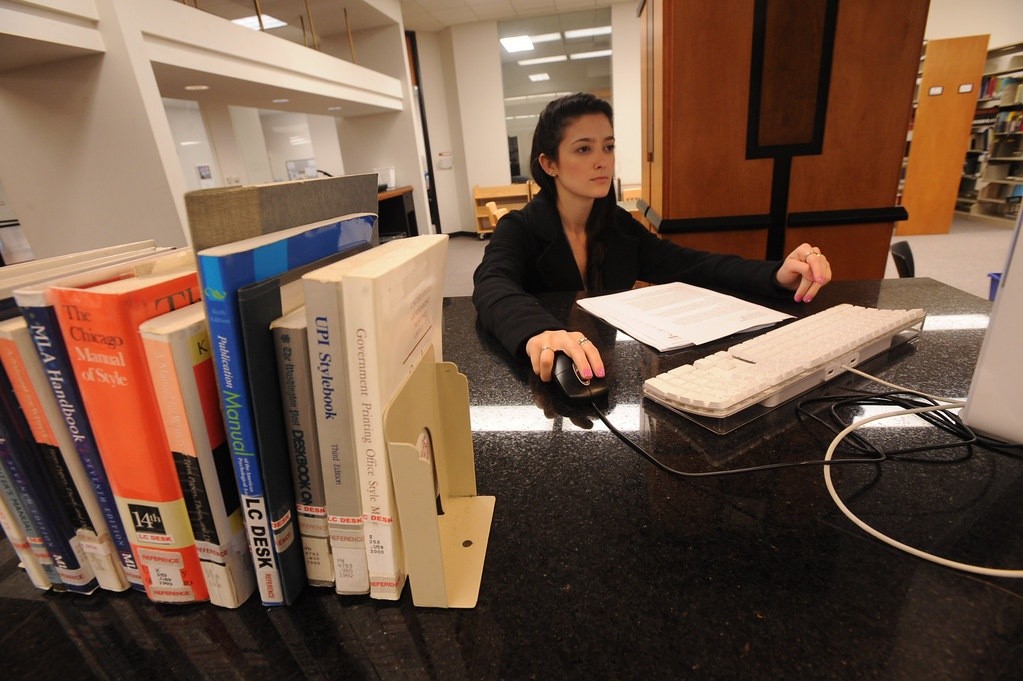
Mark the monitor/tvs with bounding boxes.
[963,198,1023,447]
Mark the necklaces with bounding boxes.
[472,92,832,382]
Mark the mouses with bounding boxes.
[549,349,608,407]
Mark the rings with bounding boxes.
[578,337,589,346]
[804,251,819,263]
[540,347,555,354]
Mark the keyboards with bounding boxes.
[644,303,928,435]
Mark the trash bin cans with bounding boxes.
[987,272,1003,301]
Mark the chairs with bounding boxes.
[891,241,915,278]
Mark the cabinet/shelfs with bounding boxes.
[893,34,990,236]
[471,180,531,241]
[954,42,1023,230]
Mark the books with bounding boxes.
[0,172,449,609]
[960,76,1023,213]
[46,558,511,681]
